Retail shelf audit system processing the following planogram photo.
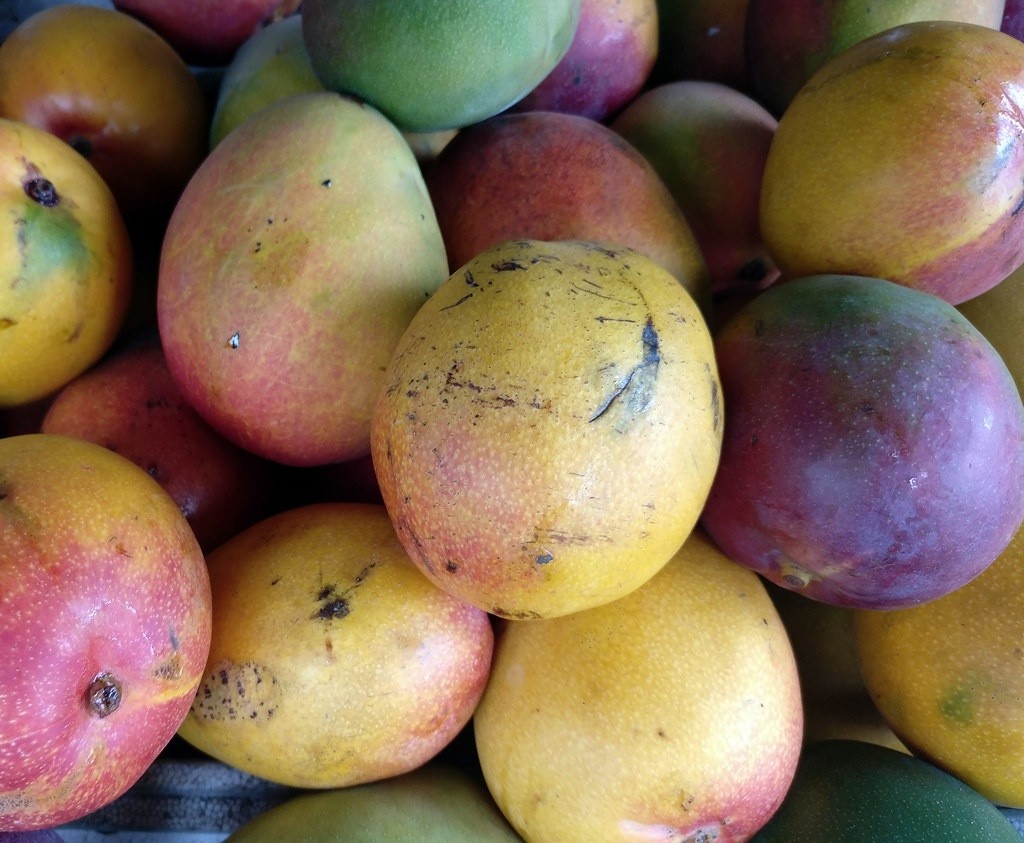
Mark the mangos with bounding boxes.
[0,0,1024,843]
